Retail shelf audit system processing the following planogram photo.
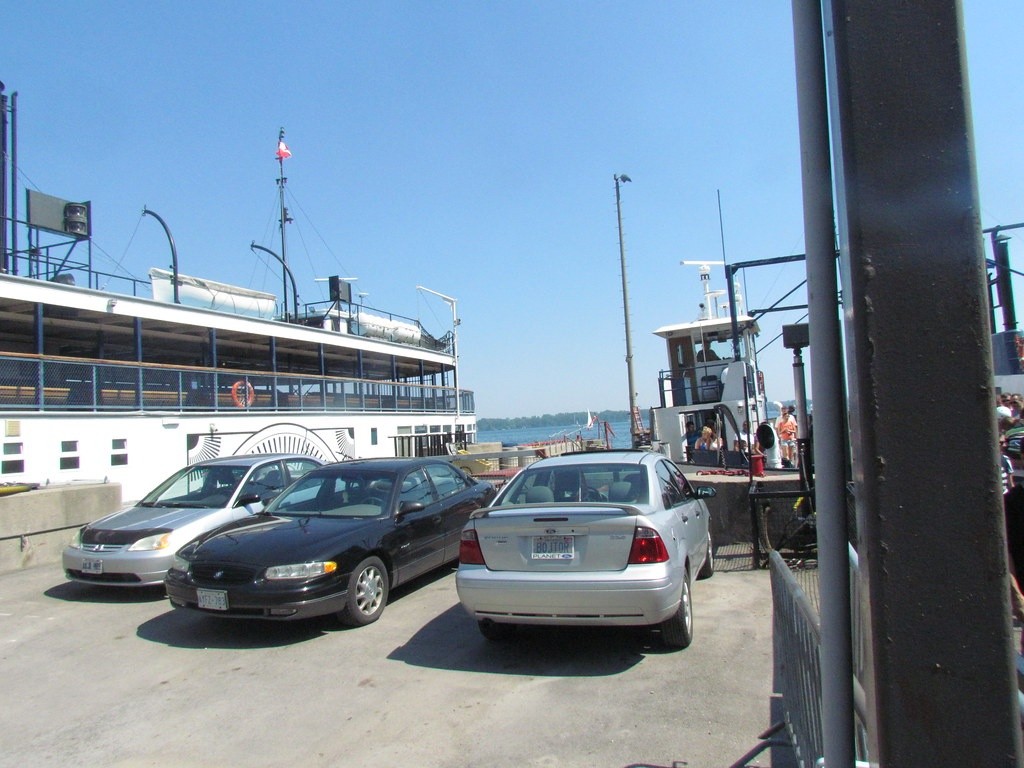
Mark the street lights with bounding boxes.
[613,172,638,446]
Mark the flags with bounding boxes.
[275,137,291,165]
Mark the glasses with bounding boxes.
[999,441,1008,446]
[1001,400,1010,402]
[1010,401,1017,405]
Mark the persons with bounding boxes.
[696,340,722,362]
[995,392,1024,500]
[684,405,799,468]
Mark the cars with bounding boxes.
[455,447,716,654]
[62,454,495,621]
[1001,427,1024,461]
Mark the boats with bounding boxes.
[0,82,477,495]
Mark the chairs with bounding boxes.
[527,486,554,502]
[607,481,640,505]
